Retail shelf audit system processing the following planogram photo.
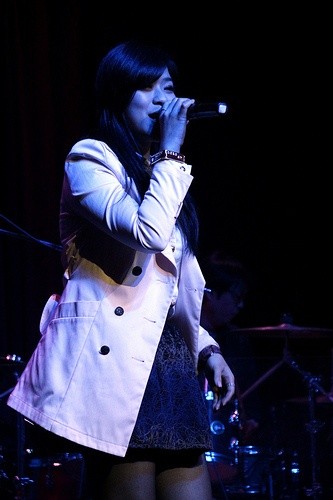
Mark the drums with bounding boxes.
[197,391,246,481]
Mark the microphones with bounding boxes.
[186,103,229,120]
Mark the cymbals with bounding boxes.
[230,317,329,333]
[288,390,333,404]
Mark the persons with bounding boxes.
[8,43,236,500]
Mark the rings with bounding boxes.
[162,108,166,111]
[226,382,235,387]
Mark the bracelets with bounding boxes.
[150,150,185,164]
